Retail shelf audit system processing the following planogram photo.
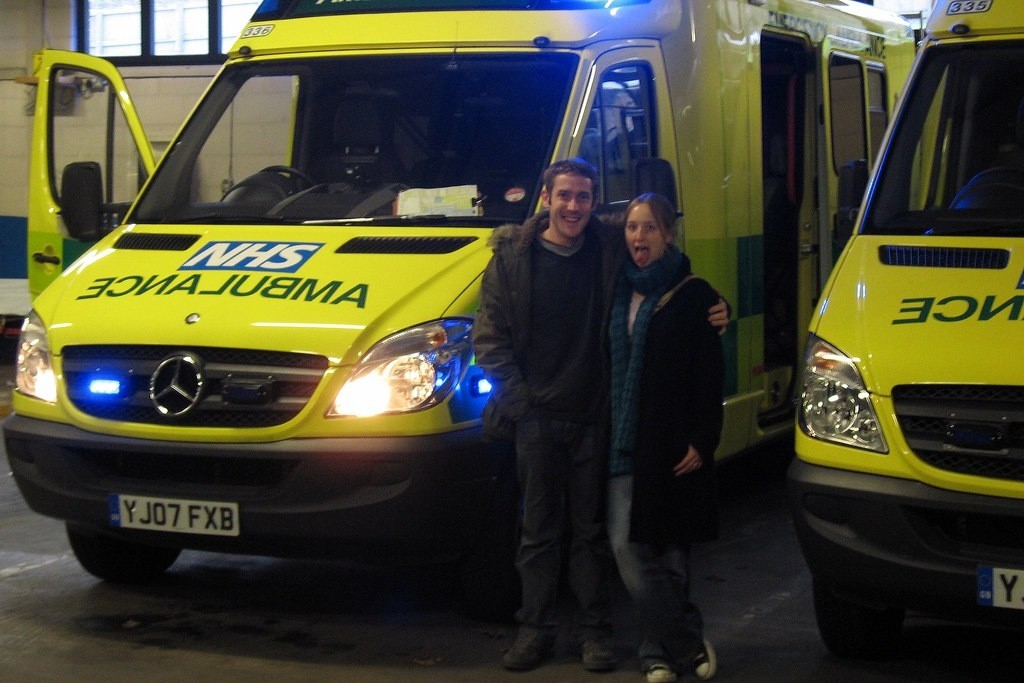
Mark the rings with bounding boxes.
[693,462,697,466]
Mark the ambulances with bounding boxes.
[787,1,1023,663]
[2,1,952,620]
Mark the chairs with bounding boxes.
[323,100,402,180]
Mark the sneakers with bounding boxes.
[691,639,717,681]
[645,663,676,683]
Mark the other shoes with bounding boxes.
[503,627,558,671]
[582,638,618,672]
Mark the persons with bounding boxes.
[473,159,733,683]
[606,192,723,683]
